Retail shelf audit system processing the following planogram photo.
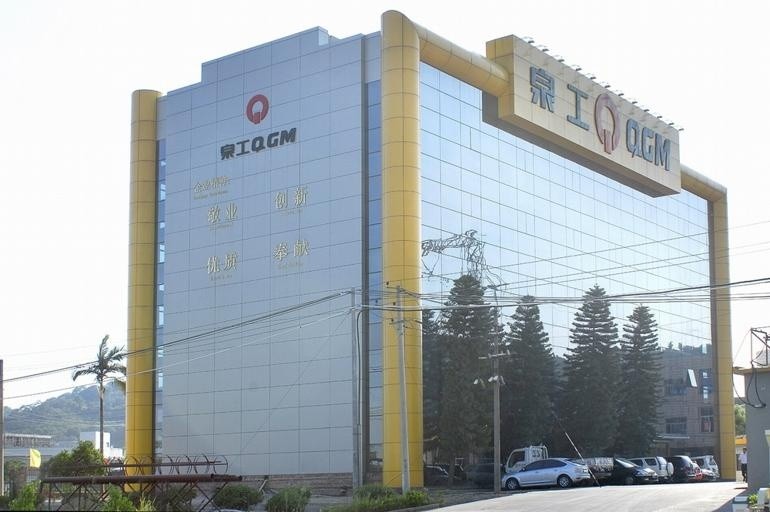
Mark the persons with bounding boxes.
[738,448,746,481]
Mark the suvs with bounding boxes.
[628,456,675,481]
[665,455,697,480]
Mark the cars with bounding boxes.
[500,457,589,490]
[692,455,718,480]
[614,457,659,484]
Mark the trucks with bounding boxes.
[504,445,613,485]
